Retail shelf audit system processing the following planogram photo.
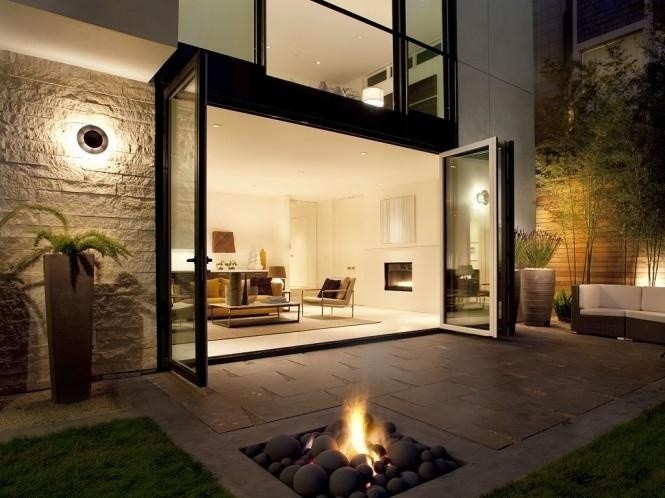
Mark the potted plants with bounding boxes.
[1,200,134,405]
[516,228,564,327]
[553,291,574,323]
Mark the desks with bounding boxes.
[281,291,291,312]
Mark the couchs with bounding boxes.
[183,281,286,320]
[571,283,665,345]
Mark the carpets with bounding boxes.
[171,312,382,345]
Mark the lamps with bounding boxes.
[363,87,385,108]
[269,267,286,290]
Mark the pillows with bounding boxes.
[207,276,273,297]
[335,278,350,300]
[317,278,342,299]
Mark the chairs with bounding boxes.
[301,277,356,317]
[170,278,194,332]
[453,265,489,310]
